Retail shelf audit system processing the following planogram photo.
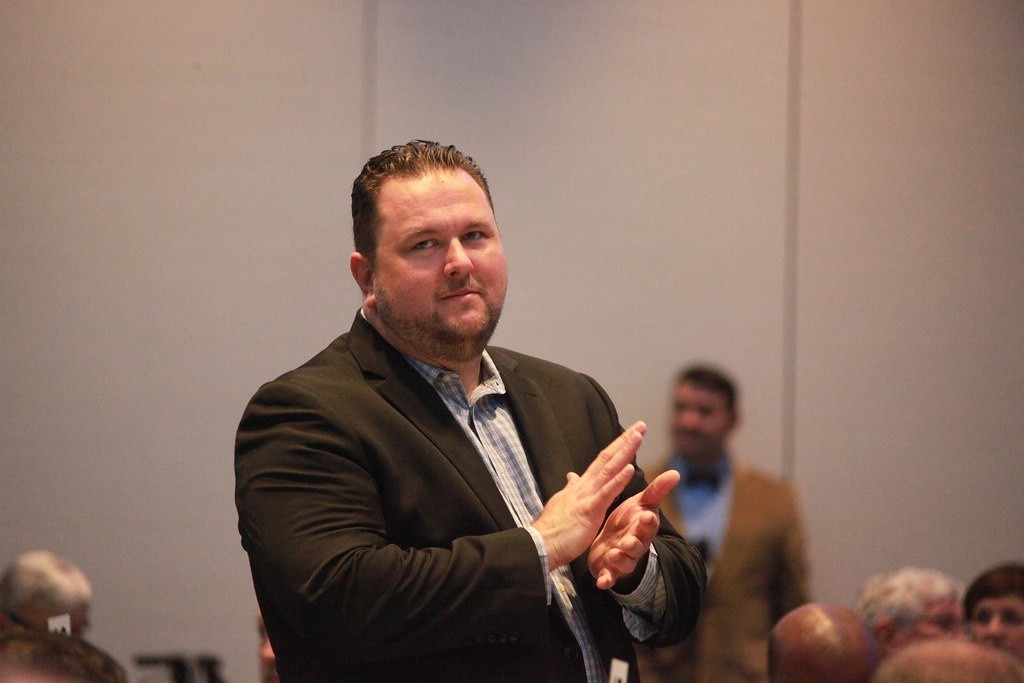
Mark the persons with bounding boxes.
[642,364,1024,683]
[0,550,126,683]
[234,141,708,683]
